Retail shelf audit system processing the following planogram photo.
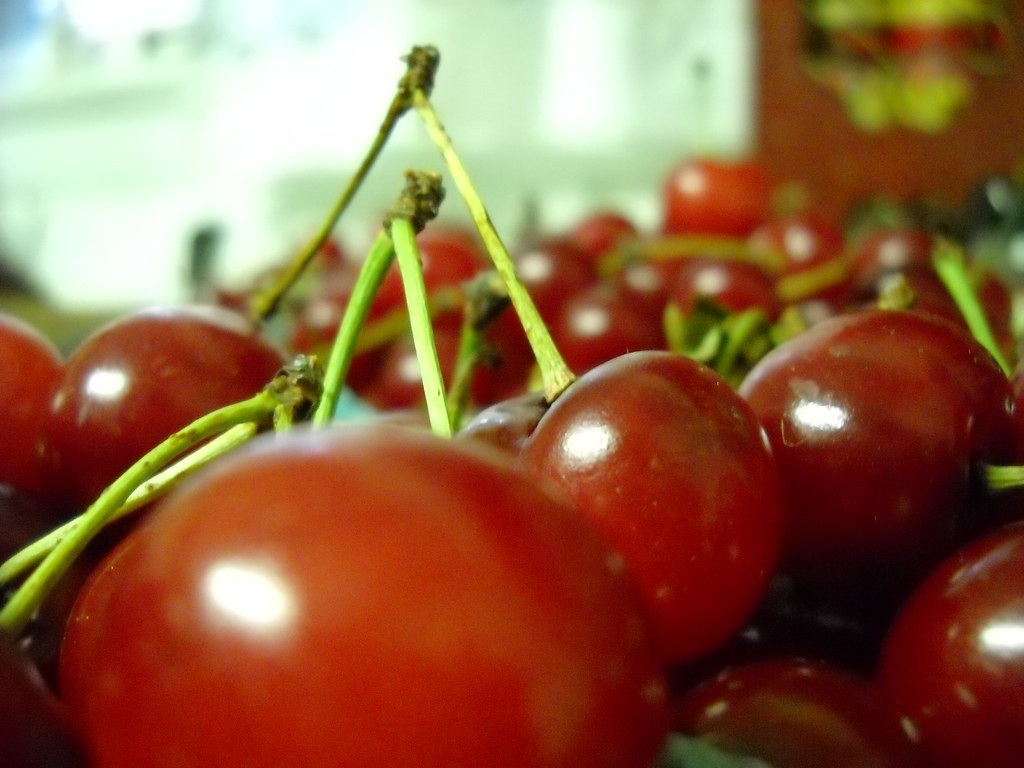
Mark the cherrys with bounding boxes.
[0,0,1024,768]
